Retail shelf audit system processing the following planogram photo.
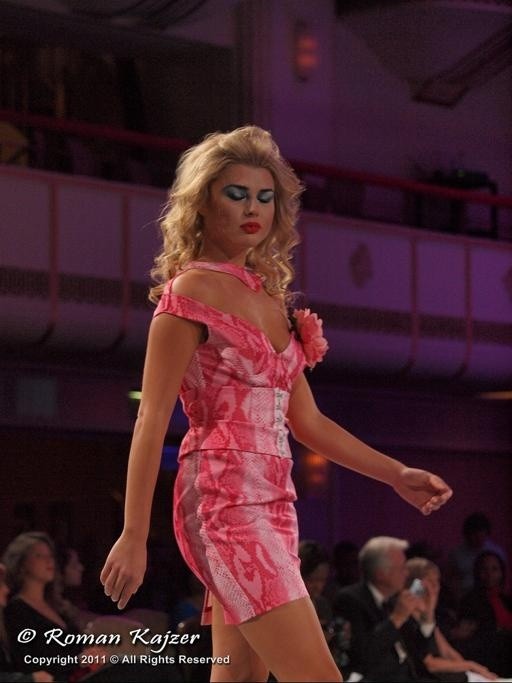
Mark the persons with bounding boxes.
[98,123,457,682]
[2,506,512,682]
